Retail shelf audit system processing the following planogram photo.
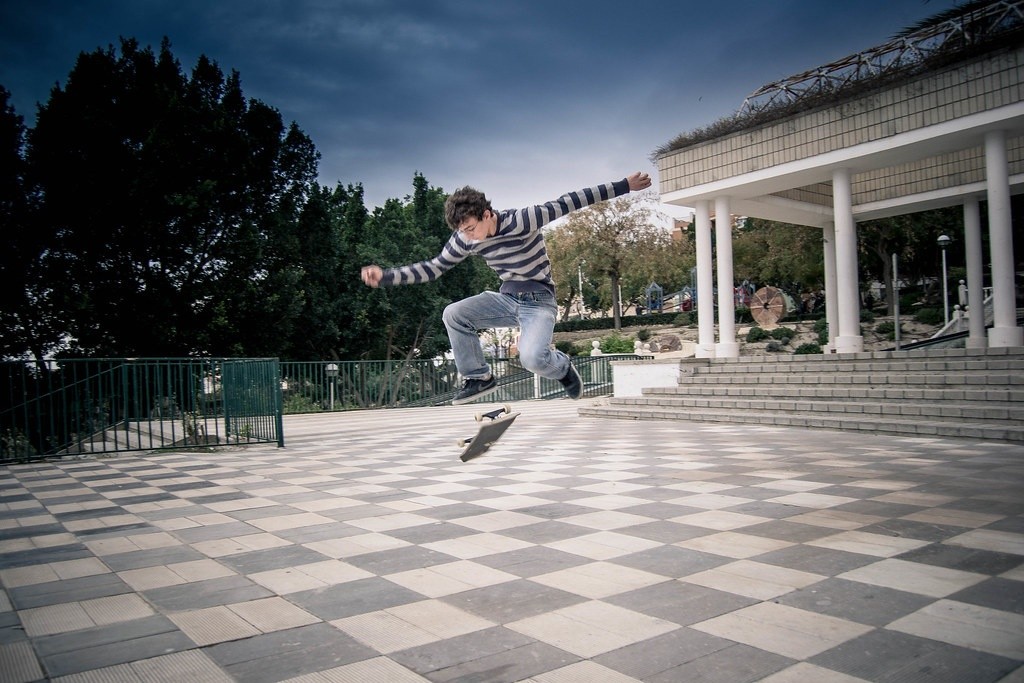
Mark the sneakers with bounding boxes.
[452,375,499,406]
[558,362,583,401]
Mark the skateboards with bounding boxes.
[459,404,522,462]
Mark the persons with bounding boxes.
[635,304,648,315]
[361,171,652,406]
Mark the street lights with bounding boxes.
[617,277,624,317]
[936,233,951,326]
[325,363,341,410]
[578,258,587,320]
